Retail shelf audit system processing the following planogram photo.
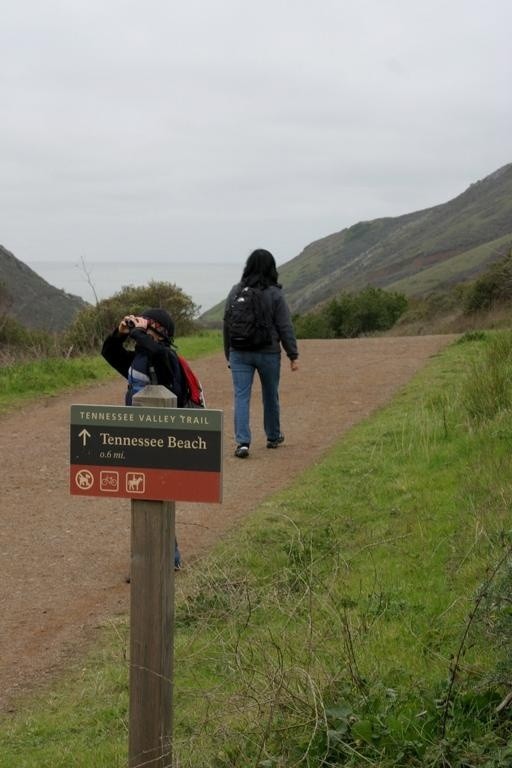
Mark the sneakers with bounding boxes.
[267,432,284,448]
[234,445,249,457]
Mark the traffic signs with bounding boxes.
[64,401,225,504]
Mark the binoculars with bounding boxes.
[124,319,135,328]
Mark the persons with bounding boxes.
[101,309,205,583]
[223,249,299,458]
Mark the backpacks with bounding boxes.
[228,283,274,347]
[146,346,206,409]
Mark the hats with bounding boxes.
[140,308,179,348]
